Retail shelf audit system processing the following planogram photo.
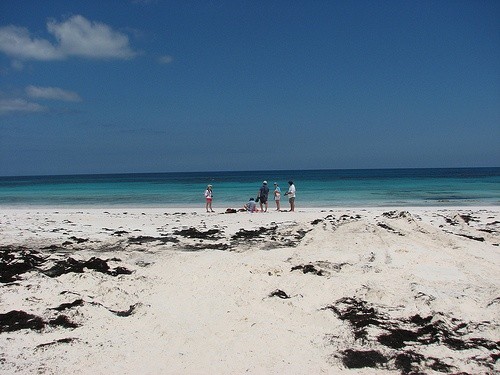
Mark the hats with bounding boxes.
[287,180,294,184]
[263,181,267,184]
[274,183,278,185]
[208,184,212,187]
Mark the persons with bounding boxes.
[284,181,296,212]
[244,198,257,211]
[273,182,281,211]
[204,185,215,213]
[258,181,269,213]
